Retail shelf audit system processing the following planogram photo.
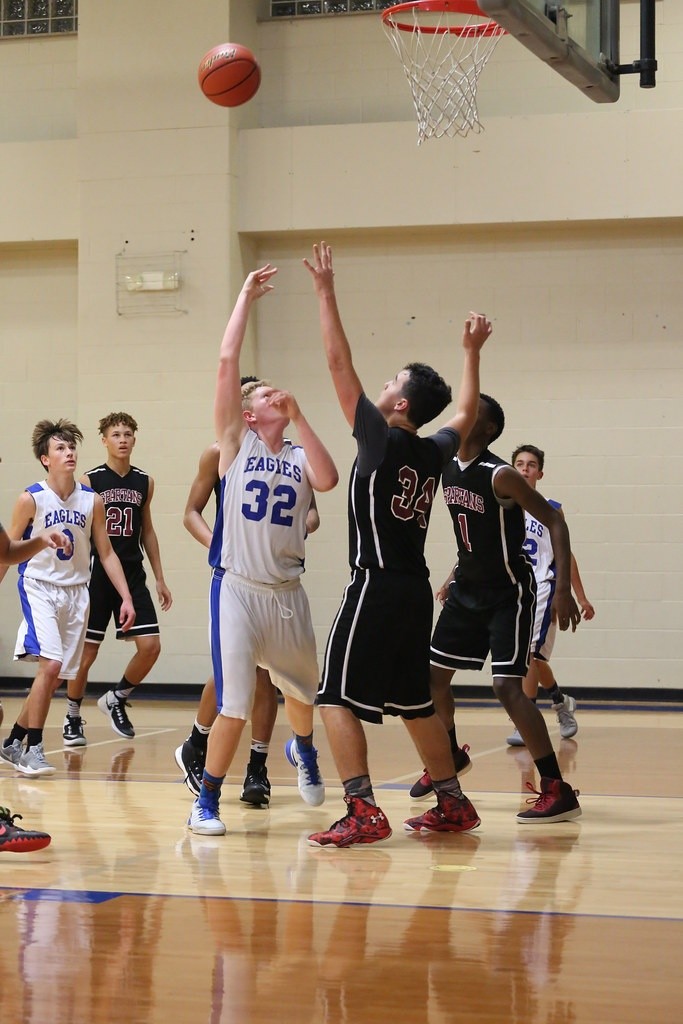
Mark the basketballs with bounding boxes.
[197,42,262,107]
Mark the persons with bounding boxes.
[301,241,494,849]
[0,418,136,778]
[174,375,320,809]
[408,392,582,824]
[0,456,75,853]
[186,263,339,836]
[505,444,596,747]
[62,412,173,747]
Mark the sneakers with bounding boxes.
[97,688,136,740]
[174,734,222,799]
[550,694,577,739]
[63,714,86,746]
[307,794,393,849]
[286,739,325,808]
[187,798,227,836]
[506,729,524,746]
[516,777,582,824]
[409,748,474,802]
[0,806,52,853]
[0,738,57,778]
[240,763,272,809]
[403,791,482,832]
